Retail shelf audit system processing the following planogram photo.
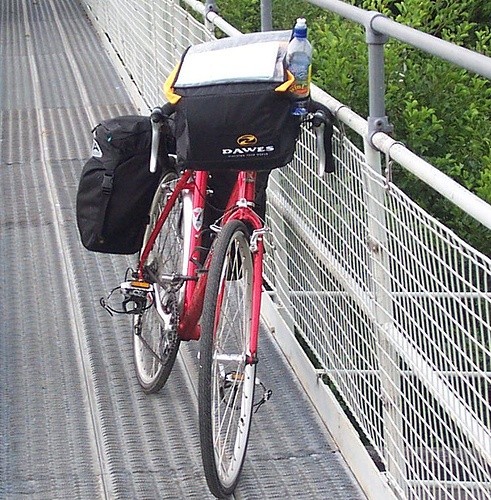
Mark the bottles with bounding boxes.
[287,17,313,114]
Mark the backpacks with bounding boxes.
[76,115,177,254]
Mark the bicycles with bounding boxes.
[98,97,335,498]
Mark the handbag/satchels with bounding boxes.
[162,29,335,171]
